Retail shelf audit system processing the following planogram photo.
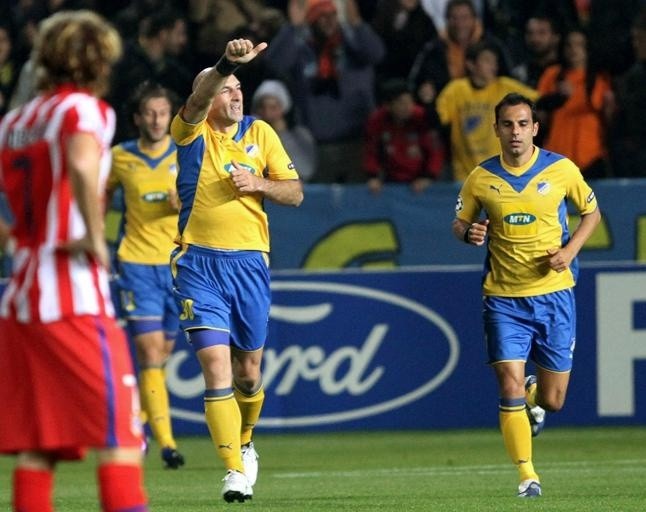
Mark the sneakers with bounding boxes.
[517,479,543,498]
[221,439,258,501]
[525,375,546,435]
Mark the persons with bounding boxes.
[453,91,601,498]
[170,37,304,499]
[1,11,153,510]
[105,77,186,467]
[1,0,645,193]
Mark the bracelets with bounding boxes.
[464,227,470,244]
[216,56,239,76]
